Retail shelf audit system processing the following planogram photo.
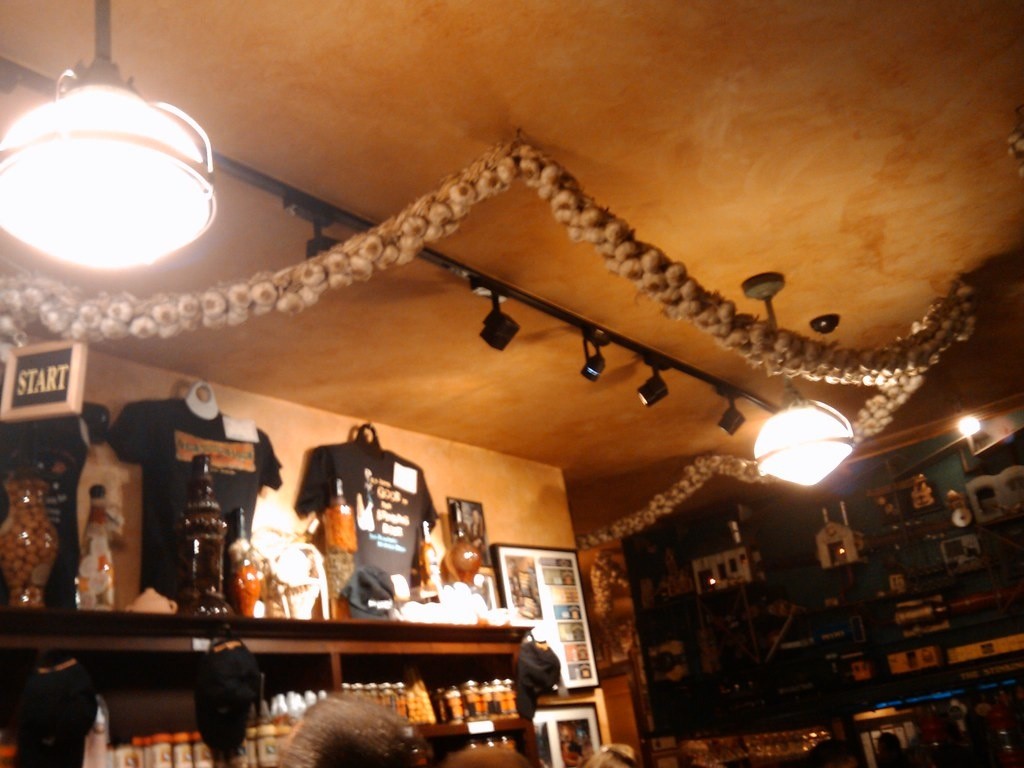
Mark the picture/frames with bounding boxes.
[491,544,600,694]
[529,701,603,768]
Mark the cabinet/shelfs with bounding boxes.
[0,623,533,768]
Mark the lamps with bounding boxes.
[582,325,610,383]
[714,386,745,435]
[281,200,341,260]
[471,279,519,351]
[742,271,855,488]
[0,0,219,270]
[638,355,672,406]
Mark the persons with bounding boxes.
[585,743,640,768]
[275,693,421,768]
[812,740,862,767]
[930,709,1003,768]
[877,733,914,768]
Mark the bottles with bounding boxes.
[429,678,520,752]
[417,519,439,587]
[322,477,356,620]
[177,453,266,617]
[0,469,58,607]
[108,687,326,768]
[341,681,406,717]
[443,501,481,594]
[73,485,115,612]
